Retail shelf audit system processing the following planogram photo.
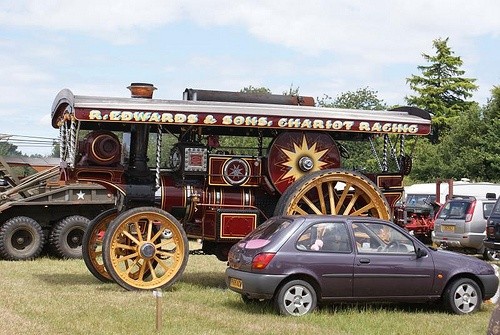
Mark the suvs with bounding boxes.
[395,193,500,261]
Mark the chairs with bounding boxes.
[322,229,351,252]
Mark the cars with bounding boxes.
[224,214,499,318]
